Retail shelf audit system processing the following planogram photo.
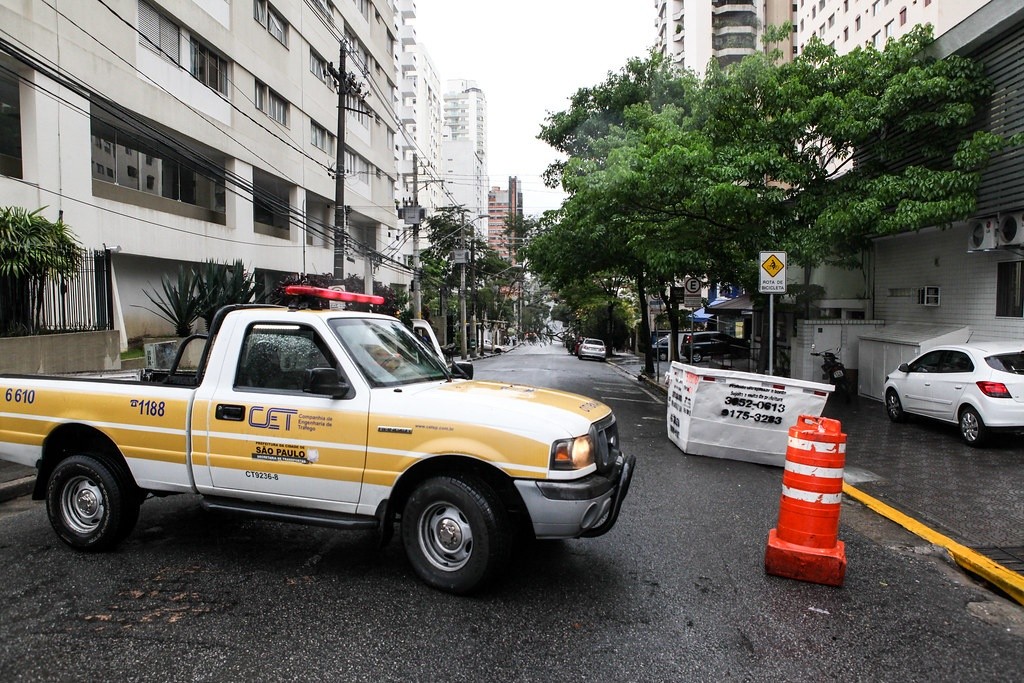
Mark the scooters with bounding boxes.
[809,342,853,404]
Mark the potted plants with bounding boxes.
[129,266,211,370]
[0,205,121,378]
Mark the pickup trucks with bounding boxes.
[1,283,638,595]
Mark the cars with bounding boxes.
[562,333,585,356]
[578,337,608,362]
[882,342,1024,447]
[651,337,669,361]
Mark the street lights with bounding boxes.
[461,263,521,359]
[411,213,492,335]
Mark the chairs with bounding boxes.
[246,340,282,390]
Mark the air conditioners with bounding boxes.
[965,215,998,252]
[997,211,1024,246]
[913,286,940,307]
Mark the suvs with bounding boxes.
[680,331,751,364]
